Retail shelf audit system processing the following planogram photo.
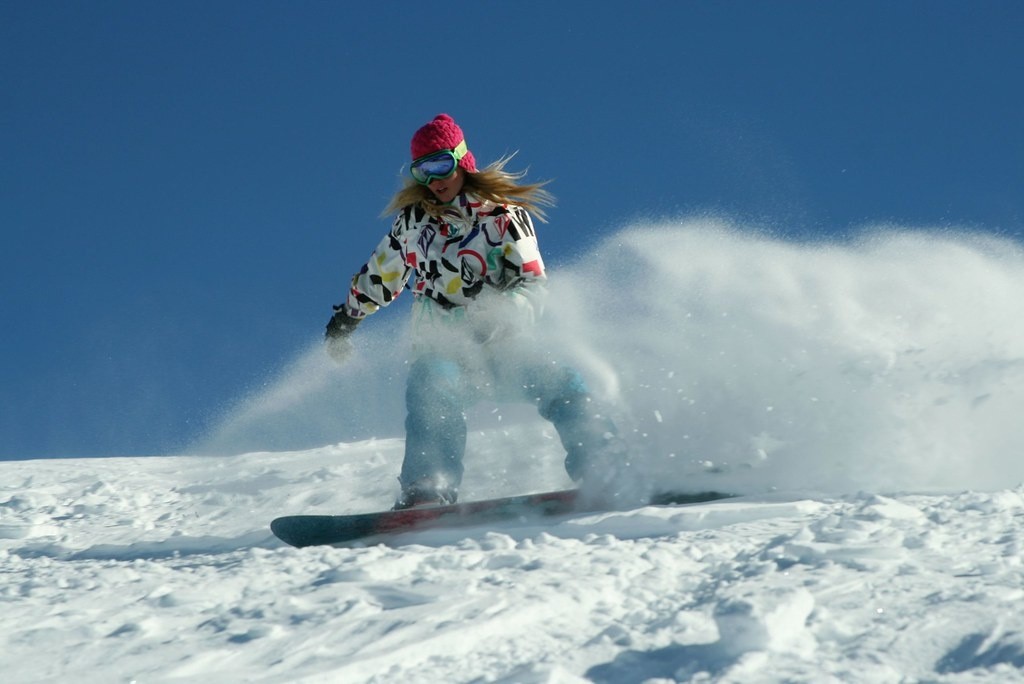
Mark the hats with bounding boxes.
[411,114,476,173]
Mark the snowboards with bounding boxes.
[268,461,748,550]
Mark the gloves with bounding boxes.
[325,316,354,364]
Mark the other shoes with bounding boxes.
[392,493,444,511]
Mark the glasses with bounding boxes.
[410,140,468,185]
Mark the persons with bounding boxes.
[324,113,636,519]
